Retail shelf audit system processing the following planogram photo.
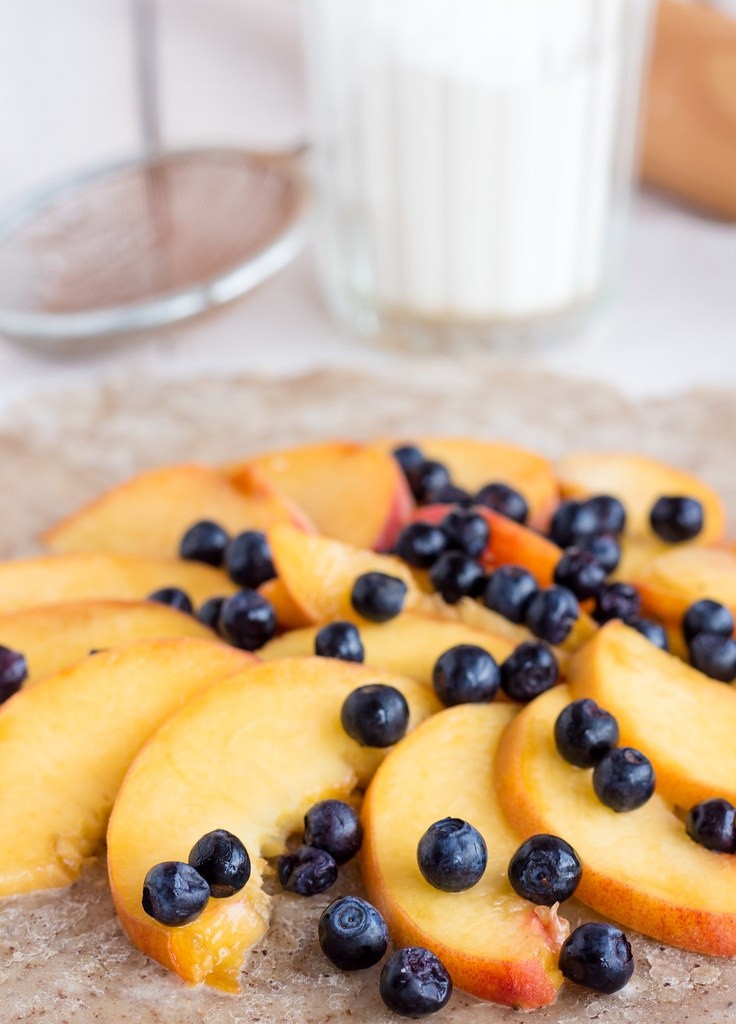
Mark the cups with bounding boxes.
[0,0,309,334]
[302,0,650,351]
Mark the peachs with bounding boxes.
[0,432,736,1012]
